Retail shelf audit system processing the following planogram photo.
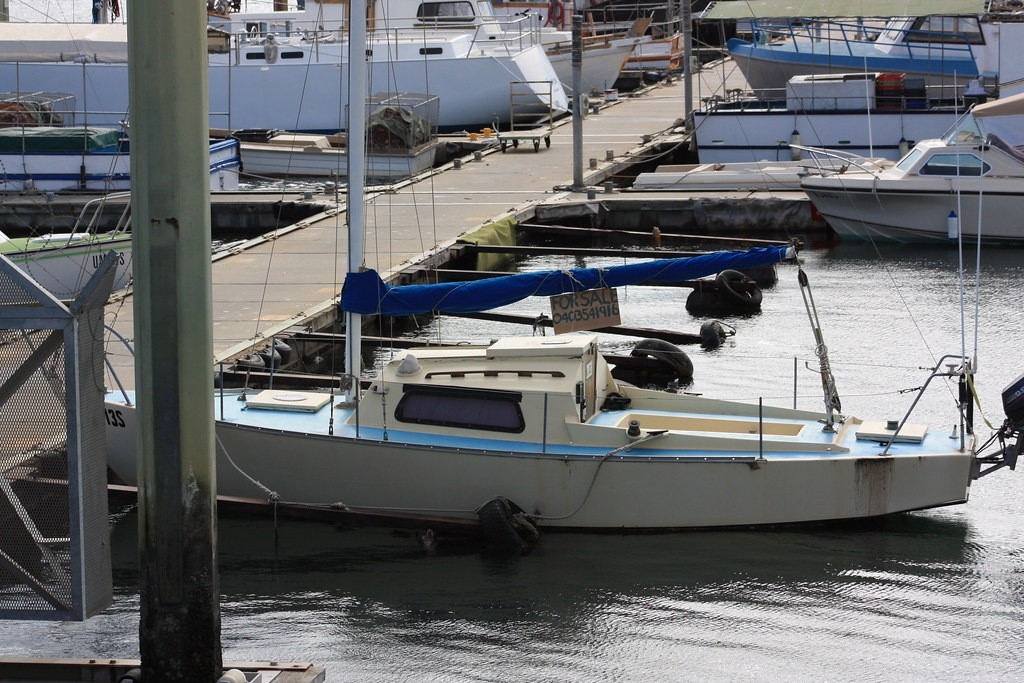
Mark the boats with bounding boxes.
[2,0,1024,188]
[0,227,133,295]
[789,87,1021,243]
[40,0,1023,529]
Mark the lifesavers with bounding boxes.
[476,494,541,555]
[717,267,763,309]
[630,337,693,381]
[700,319,727,346]
